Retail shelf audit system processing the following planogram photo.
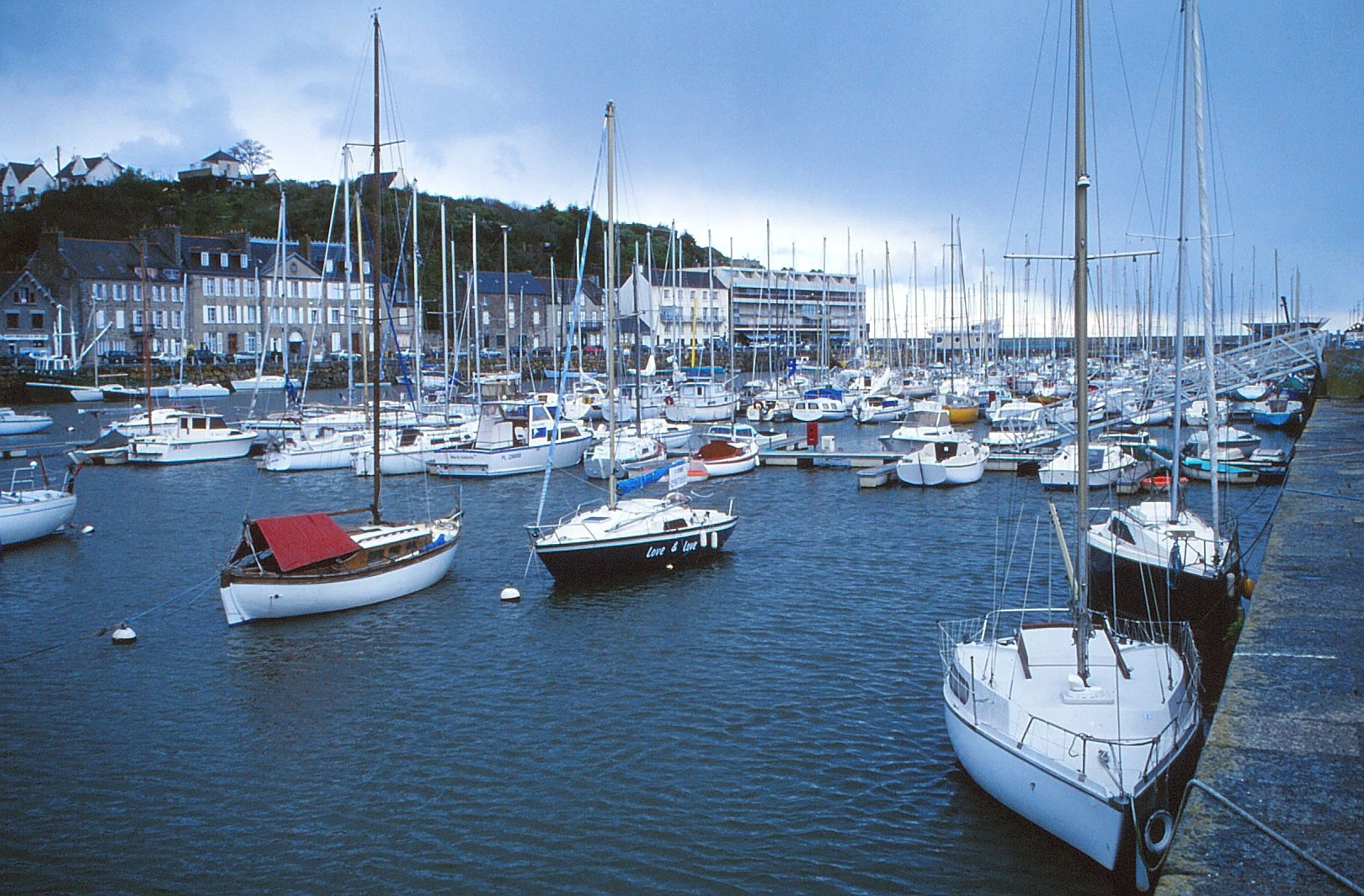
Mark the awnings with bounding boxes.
[743,332,780,341]
[818,335,851,341]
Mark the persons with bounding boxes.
[482,399,493,418]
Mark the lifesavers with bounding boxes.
[582,395,591,404]
[665,395,674,405]
[753,401,763,409]
[669,494,688,508]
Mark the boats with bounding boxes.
[0,442,86,547]
[0,404,55,436]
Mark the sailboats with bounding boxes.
[26,169,1315,493]
[519,97,739,582]
[214,6,467,628]
[1087,0,1241,621]
[935,0,1211,876]
